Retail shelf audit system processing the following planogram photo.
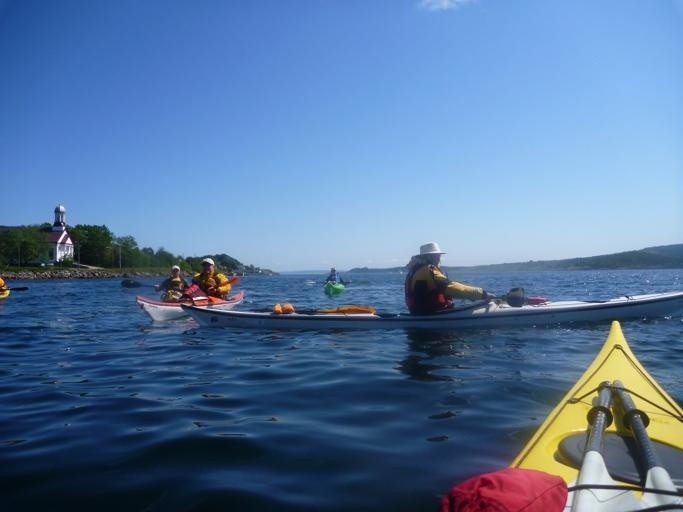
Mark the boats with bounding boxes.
[134,290,243,323]
[0,277,12,299]
[323,282,344,295]
[180,289,682,331]
[506,318,682,512]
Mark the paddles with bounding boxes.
[206,278,239,292]
[305,280,353,286]
[251,306,375,314]
[1,287,29,291]
[564,382,683,512]
[490,288,525,307]
[121,279,158,289]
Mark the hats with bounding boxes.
[199,258,215,264]
[172,265,180,272]
[414,242,447,257]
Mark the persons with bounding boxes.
[192,258,231,299]
[154,265,189,294]
[405,242,497,314]
[326,267,344,283]
